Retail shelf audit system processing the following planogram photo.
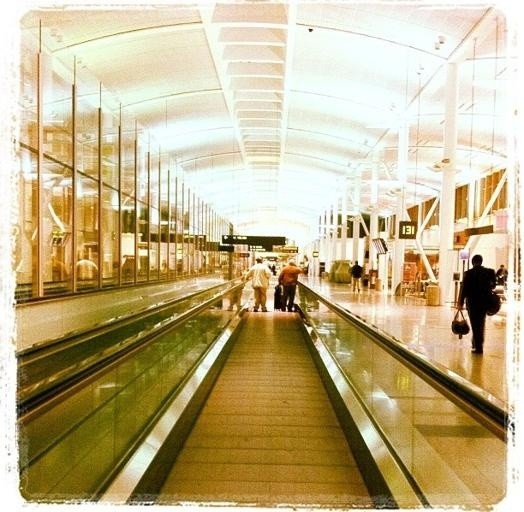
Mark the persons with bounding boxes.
[494,264,508,286]
[44,252,182,284]
[225,287,244,313]
[298,284,313,309]
[348,260,363,294]
[276,259,304,311]
[243,257,273,313]
[455,253,497,356]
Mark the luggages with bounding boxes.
[273,285,282,310]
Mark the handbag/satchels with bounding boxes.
[486,294,500,315]
[452,320,469,335]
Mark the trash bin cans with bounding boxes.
[322,272,328,279]
[426,286,439,306]
[375,278,383,291]
